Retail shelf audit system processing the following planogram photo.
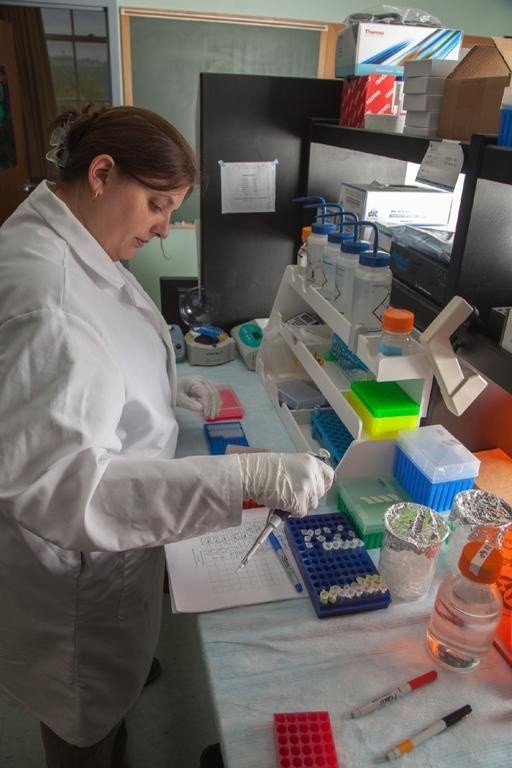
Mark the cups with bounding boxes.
[448,488,512,575]
[379,500,451,601]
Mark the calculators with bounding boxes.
[285,312,318,330]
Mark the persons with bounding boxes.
[0,104,335,768]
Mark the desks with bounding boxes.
[174,358,512,768]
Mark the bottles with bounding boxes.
[377,308,414,356]
[425,540,505,675]
[305,221,393,333]
[296,226,312,269]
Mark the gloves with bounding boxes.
[176,376,222,420]
[239,452,335,519]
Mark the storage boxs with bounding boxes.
[334,23,512,142]
[340,182,454,240]
[395,424,482,512]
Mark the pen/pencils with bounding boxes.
[268,530,304,594]
[350,670,438,721]
[381,704,473,767]
